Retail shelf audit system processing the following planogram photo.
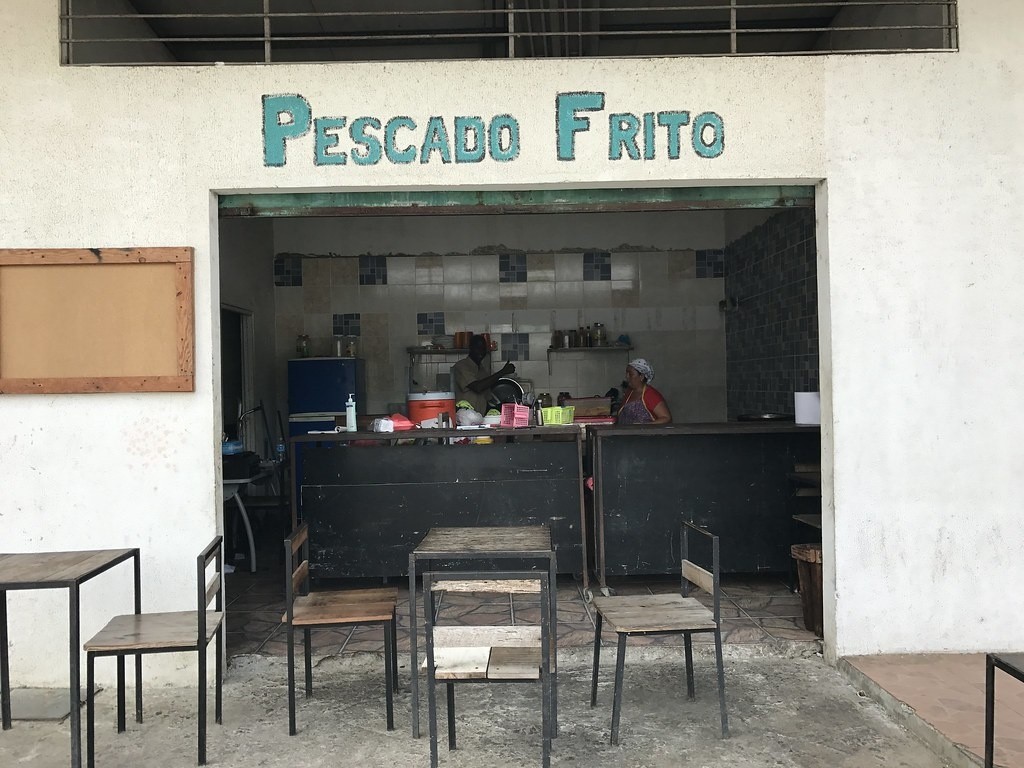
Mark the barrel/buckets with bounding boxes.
[407,392,456,429]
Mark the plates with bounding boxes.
[431,335,455,348]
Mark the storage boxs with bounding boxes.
[561,396,616,441]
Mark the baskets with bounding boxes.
[501,403,529,428]
[541,406,575,424]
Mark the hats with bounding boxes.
[628,358,655,384]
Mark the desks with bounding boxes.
[0,547,143,768]
[408,524,558,740]
[223,467,268,576]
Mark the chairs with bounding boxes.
[284,524,399,736]
[590,520,730,746]
[422,569,551,768]
[83,535,224,768]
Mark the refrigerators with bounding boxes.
[288,357,365,532]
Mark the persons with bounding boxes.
[618,358,672,424]
[451,334,516,418]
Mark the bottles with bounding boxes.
[557,392,571,406]
[534,393,552,426]
[579,326,592,347]
[592,323,606,347]
[296,334,311,358]
[276,437,286,461]
[333,335,356,357]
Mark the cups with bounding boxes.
[455,332,473,349]
[481,333,497,350]
